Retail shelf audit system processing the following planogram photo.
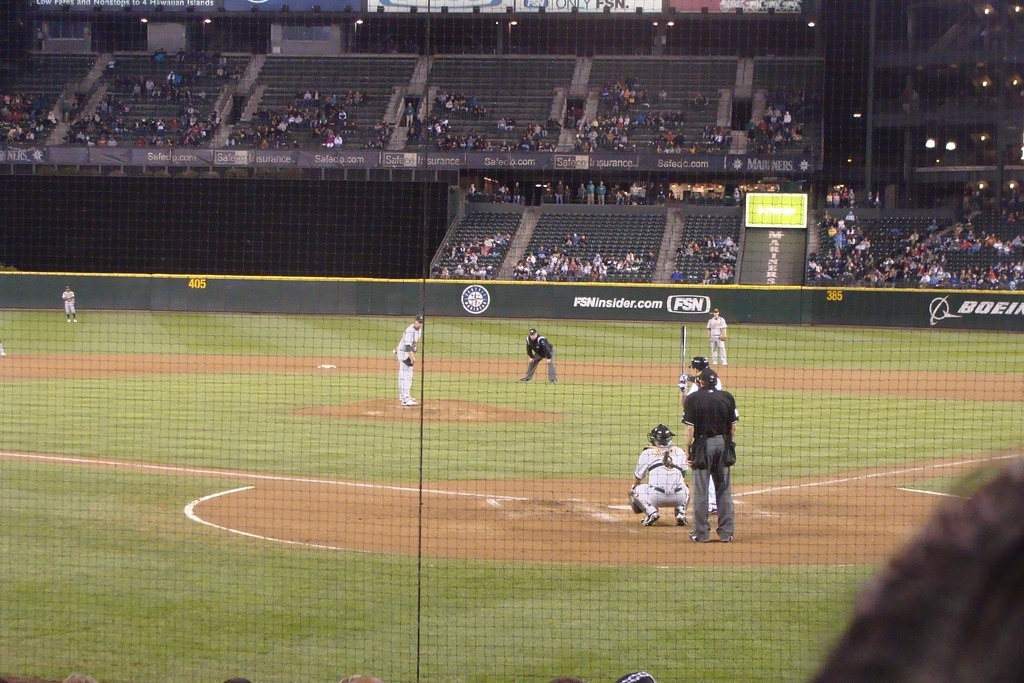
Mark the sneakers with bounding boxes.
[675,513,688,526]
[712,361,718,365]
[722,362,727,366]
[720,535,734,542]
[641,510,660,526]
[688,532,709,542]
[400,394,418,406]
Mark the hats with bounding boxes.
[714,309,719,313]
[529,329,536,335]
[415,313,424,323]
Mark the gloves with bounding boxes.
[529,359,535,363]
[678,373,687,392]
[545,359,552,365]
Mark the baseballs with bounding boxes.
[393,349,396,354]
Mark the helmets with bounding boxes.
[646,423,674,447]
[690,356,710,371]
[699,368,718,385]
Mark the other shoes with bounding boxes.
[552,380,558,384]
[520,378,529,381]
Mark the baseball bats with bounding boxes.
[681,324,686,374]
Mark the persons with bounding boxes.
[396,314,423,406]
[0,46,230,148]
[706,308,728,365]
[426,76,733,155]
[520,329,557,384]
[678,356,724,515]
[512,232,655,282]
[404,102,414,127]
[224,88,393,151]
[631,424,690,527]
[431,231,511,280]
[676,235,739,284]
[745,102,818,156]
[233,64,241,82]
[468,180,667,206]
[809,184,1024,292]
[684,369,738,543]
[61,286,78,323]
[734,187,742,208]
[0,340,6,355]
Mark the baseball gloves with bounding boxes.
[402,357,415,366]
